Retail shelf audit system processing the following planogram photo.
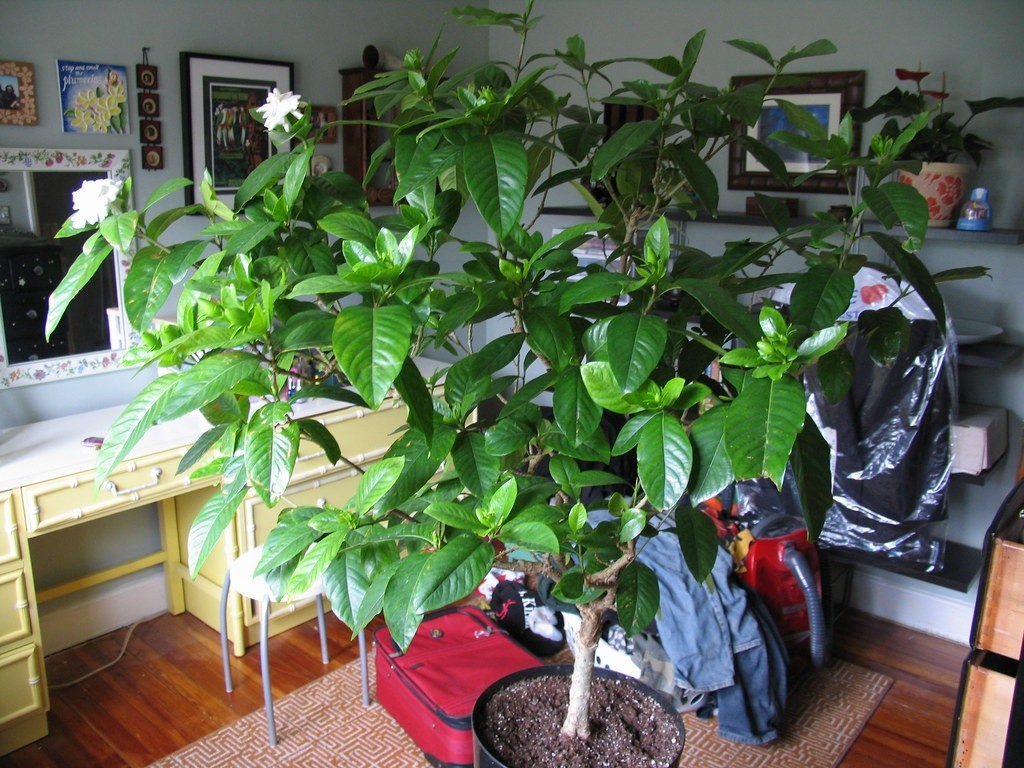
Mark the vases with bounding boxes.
[471,665,686,768]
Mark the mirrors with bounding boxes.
[0,148,151,389]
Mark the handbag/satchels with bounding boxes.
[779,261,956,574]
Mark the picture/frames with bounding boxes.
[0,60,37,125]
[727,70,865,195]
[180,52,297,217]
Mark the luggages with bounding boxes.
[376,604,546,768]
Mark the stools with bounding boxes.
[219,541,371,744]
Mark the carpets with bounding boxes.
[143,595,896,768]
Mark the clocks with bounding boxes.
[338,69,397,208]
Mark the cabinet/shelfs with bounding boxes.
[944,478,1024,768]
[537,207,1024,593]
[1,244,67,364]
[1,355,479,757]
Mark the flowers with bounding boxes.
[44,0,1024,737]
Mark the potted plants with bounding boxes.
[846,62,1024,229]
